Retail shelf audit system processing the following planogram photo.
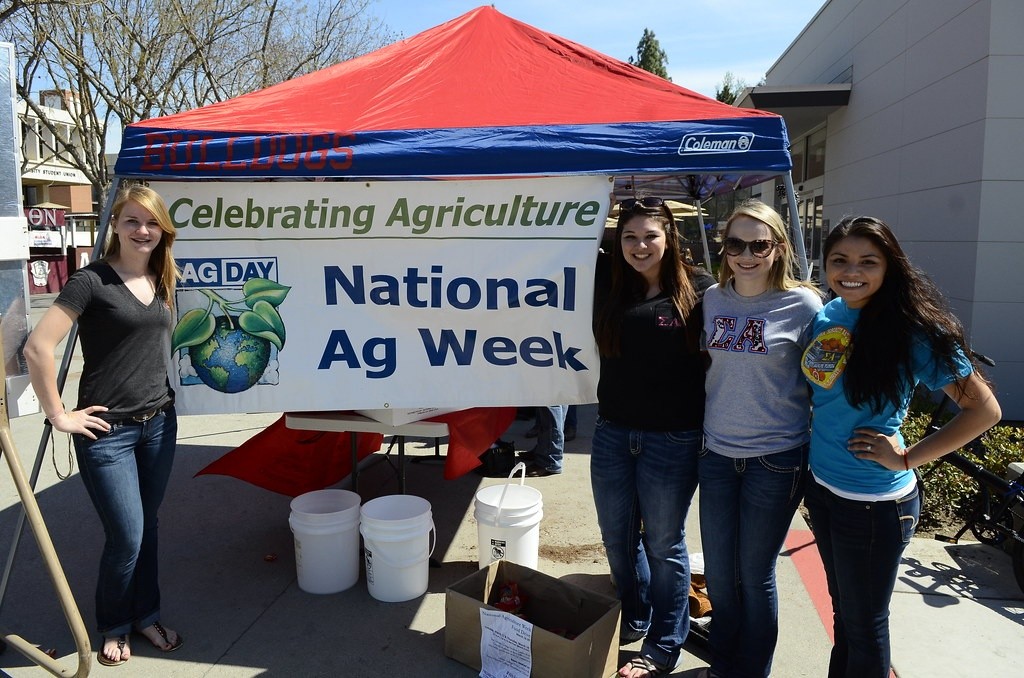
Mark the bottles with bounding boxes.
[80,252,90,268]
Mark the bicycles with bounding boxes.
[902,343,1024,594]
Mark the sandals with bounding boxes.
[97,634,132,666]
[135,622,185,652]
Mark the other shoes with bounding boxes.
[514,459,562,477]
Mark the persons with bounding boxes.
[592,196,712,678]
[700,201,825,678]
[22,185,176,666]
[518,406,577,475]
[799,214,1003,678]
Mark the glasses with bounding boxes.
[723,237,778,258]
[618,196,664,216]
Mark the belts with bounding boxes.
[108,403,174,426]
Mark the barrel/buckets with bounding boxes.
[288,488,361,596]
[358,494,438,604]
[473,463,543,570]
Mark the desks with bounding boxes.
[285,412,451,566]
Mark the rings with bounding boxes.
[867,445,871,453]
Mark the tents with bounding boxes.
[0,4,813,600]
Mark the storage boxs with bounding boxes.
[478,439,515,477]
[445,560,622,678]
[353,407,475,427]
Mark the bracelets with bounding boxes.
[45,409,64,419]
[904,449,909,471]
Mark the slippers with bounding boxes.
[615,650,682,678]
